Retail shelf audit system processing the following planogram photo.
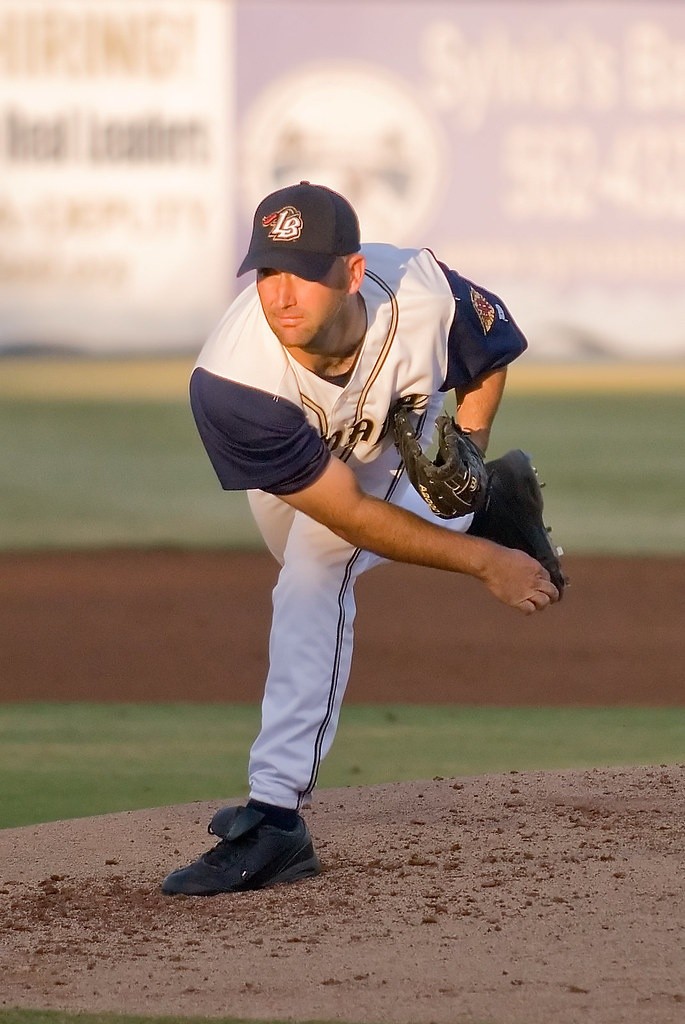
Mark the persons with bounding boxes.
[160,180,569,898]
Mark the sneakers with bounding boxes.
[162,805,323,897]
[465,448,566,595]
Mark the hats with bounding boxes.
[235,181,361,284]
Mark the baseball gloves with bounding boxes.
[391,406,490,522]
